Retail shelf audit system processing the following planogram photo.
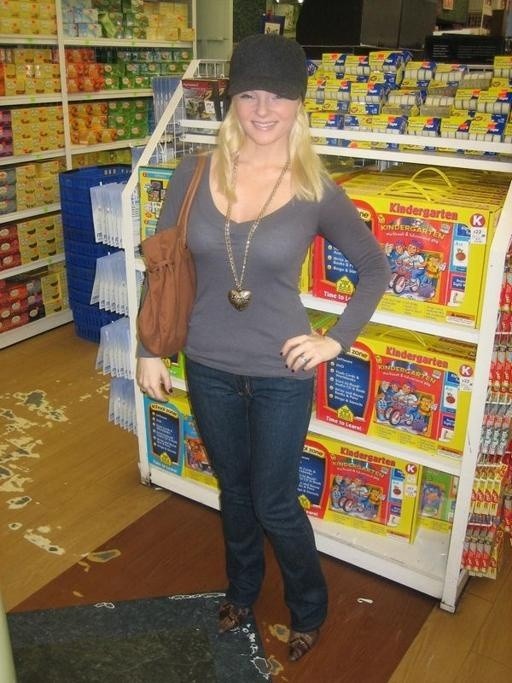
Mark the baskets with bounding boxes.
[60,164,132,342]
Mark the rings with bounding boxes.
[140,386,146,394]
[301,354,309,364]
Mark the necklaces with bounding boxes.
[223,153,292,313]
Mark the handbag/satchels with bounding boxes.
[137,226,195,357]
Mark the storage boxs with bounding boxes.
[56,161,131,342]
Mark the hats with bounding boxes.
[228,35,308,102]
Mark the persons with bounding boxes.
[133,32,393,663]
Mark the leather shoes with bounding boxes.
[218,598,249,635]
[288,628,318,661]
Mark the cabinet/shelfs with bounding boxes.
[0,1,195,350]
[124,54,511,616]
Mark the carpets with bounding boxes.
[3,587,271,681]
